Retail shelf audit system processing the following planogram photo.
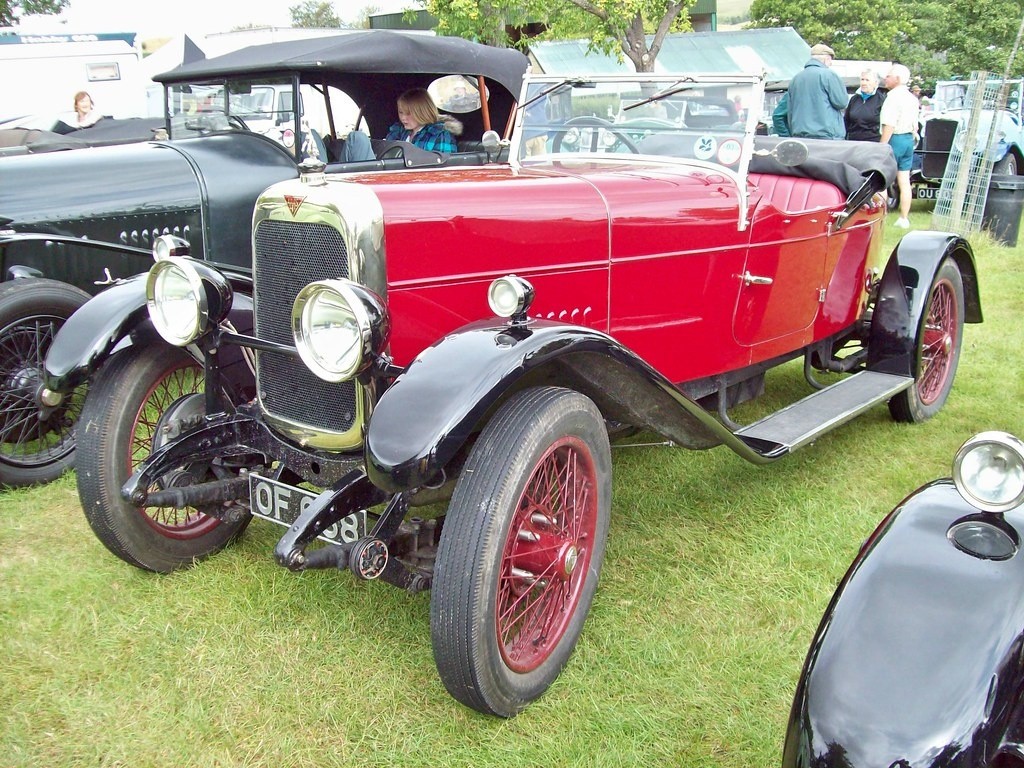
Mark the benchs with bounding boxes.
[456,140,485,152]
[747,171,846,212]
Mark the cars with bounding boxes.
[0,30,532,487]
[46,66,985,723]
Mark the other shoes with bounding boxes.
[893,217,910,229]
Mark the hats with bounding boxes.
[911,85,922,92]
[811,44,835,60]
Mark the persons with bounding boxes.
[60,90,103,129]
[384,87,458,158]
[771,40,922,230]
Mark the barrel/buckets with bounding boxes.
[971,174,1024,247]
